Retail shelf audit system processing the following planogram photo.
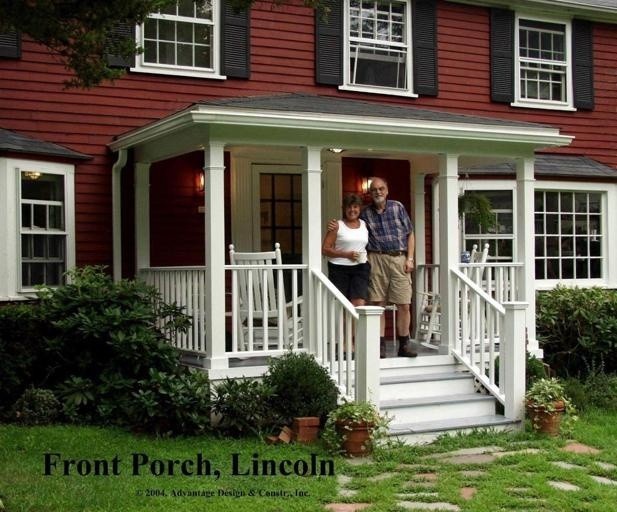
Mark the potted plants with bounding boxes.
[457,192,496,231]
[203,343,340,444]
[520,377,578,438]
[320,395,394,457]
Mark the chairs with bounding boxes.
[408,243,490,349]
[229,242,304,361]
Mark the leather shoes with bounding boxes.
[398,347,417,357]
[380,345,387,358]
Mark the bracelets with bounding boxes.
[406,256,415,262]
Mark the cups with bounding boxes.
[461,251,471,262]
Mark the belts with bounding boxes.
[368,249,407,257]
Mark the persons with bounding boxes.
[321,193,372,357]
[326,177,422,359]
